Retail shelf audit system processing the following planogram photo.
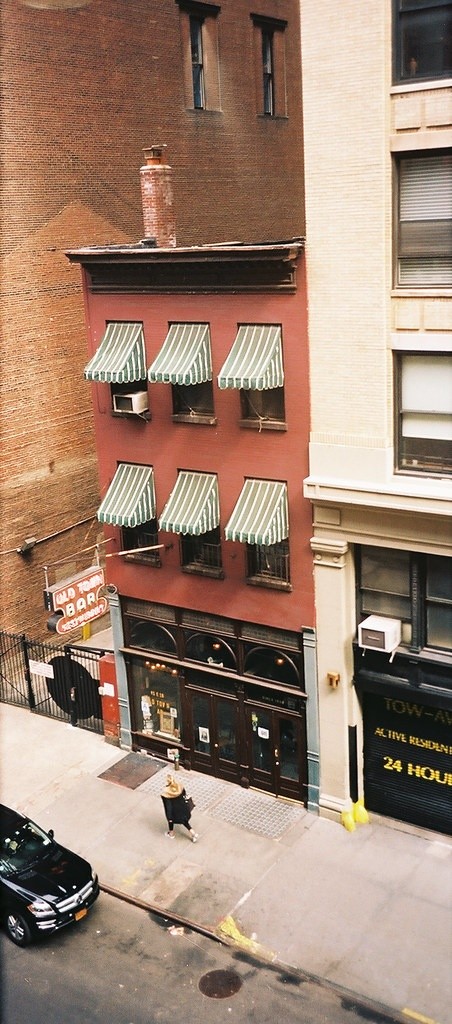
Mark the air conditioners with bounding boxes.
[357,615,402,653]
[113,392,149,414]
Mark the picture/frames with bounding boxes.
[159,711,174,733]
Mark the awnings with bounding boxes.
[94,462,290,546]
[82,321,285,392]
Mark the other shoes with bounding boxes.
[165,832,175,839]
[192,833,198,842]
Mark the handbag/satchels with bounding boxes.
[184,794,195,812]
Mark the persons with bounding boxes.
[160,773,198,843]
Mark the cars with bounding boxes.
[0,804,100,948]
